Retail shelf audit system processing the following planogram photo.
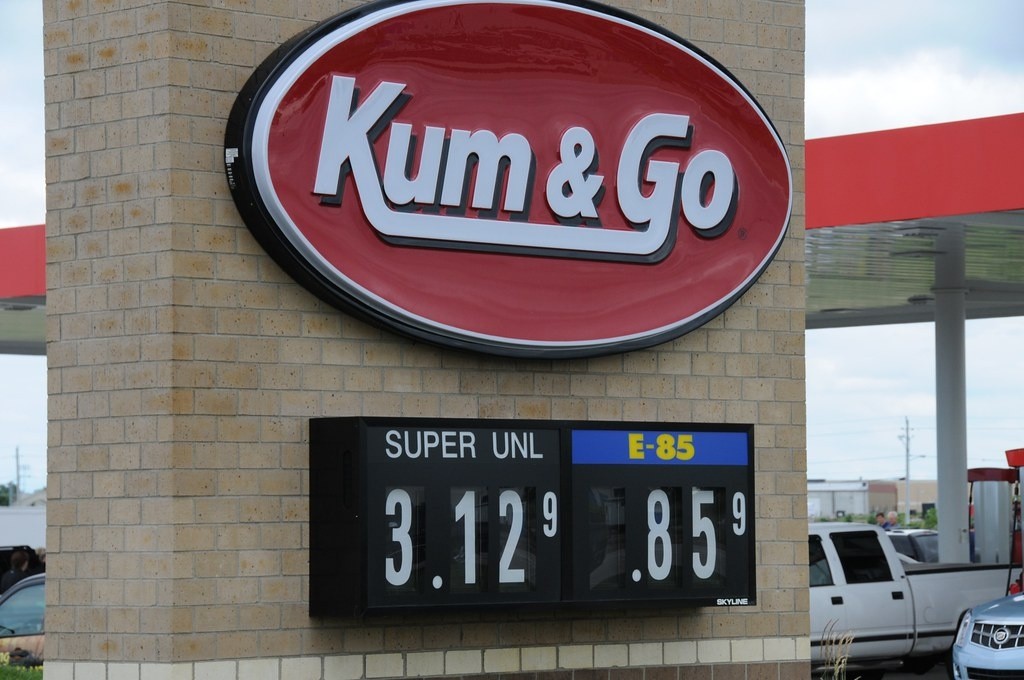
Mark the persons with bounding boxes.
[886,511,902,527]
[1,551,28,590]
[876,513,889,528]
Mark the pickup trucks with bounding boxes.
[808,520,1024,679]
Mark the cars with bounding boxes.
[952,591,1024,680]
[888,528,939,565]
[0,571,47,666]
